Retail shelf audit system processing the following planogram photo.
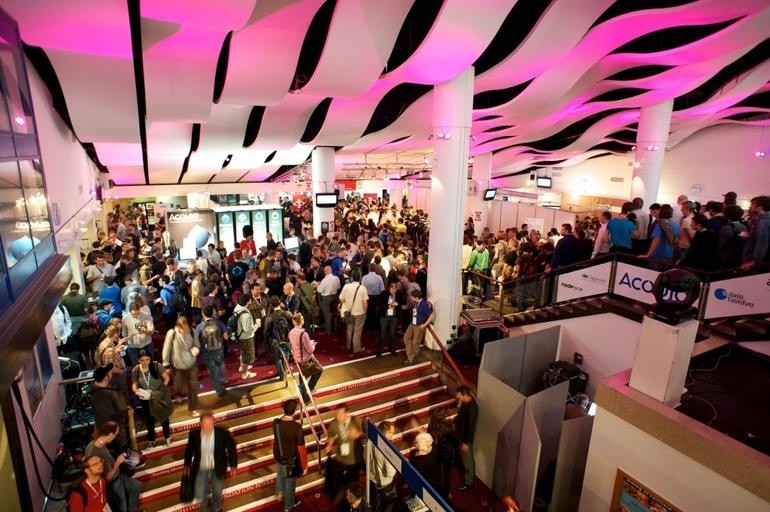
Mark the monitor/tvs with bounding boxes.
[536,177,552,188]
[484,188,497,200]
[316,193,337,206]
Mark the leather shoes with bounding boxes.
[455,483,473,491]
[375,351,383,360]
[391,350,397,356]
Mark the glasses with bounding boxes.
[84,458,105,469]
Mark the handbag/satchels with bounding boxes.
[286,454,304,478]
[298,355,325,379]
[311,303,320,317]
[343,310,352,323]
[179,463,195,502]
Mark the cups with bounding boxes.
[191,348,199,358]
[256,318,261,330]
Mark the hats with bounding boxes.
[721,191,738,198]
[92,362,114,381]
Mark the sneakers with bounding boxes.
[166,437,173,448]
[310,386,318,393]
[191,410,201,418]
[352,346,367,353]
[241,370,258,379]
[148,440,157,448]
[128,460,146,470]
[238,364,253,372]
[283,500,302,511]
[304,397,317,406]
[403,356,417,366]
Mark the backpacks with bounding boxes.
[425,298,439,326]
[201,319,223,352]
[162,282,185,313]
[65,482,88,512]
[226,309,250,345]
[268,311,290,342]
[79,312,110,345]
